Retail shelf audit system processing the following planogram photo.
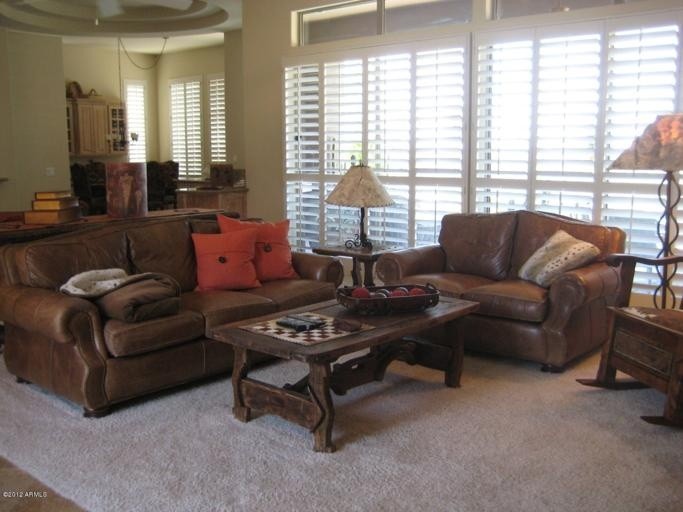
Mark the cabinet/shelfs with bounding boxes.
[66,101,76,158]
[76,102,109,158]
[108,103,129,156]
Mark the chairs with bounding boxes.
[70,160,179,212]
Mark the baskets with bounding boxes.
[335,280,441,316]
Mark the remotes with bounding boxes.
[287,315,326,326]
[277,317,305,331]
[288,314,318,330]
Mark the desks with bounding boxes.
[174,187,248,220]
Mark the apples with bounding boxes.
[352,287,424,298]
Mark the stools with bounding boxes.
[576,249,682,430]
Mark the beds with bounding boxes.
[203,295,480,453]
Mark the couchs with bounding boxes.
[375,209,628,376]
[0,212,344,419]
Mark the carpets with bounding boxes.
[0,355,680,512]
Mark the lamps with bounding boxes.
[324,166,395,251]
[605,115,683,313]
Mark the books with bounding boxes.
[23,190,83,224]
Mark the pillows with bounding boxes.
[514,228,599,290]
[188,211,303,295]
